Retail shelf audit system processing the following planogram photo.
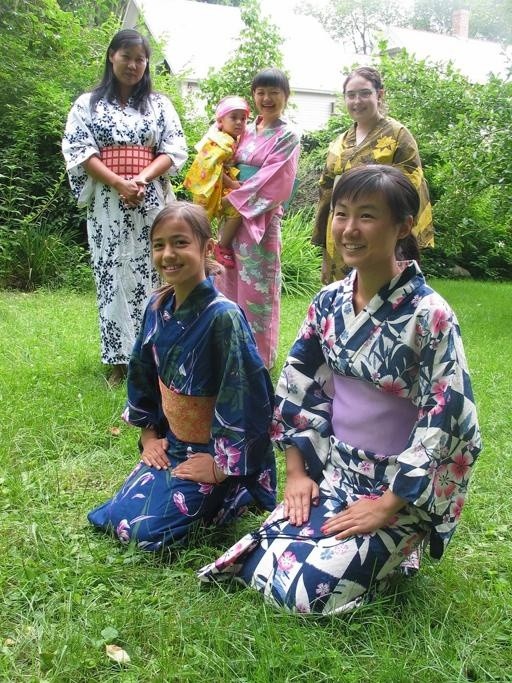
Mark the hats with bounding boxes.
[215,94,250,120]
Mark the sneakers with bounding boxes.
[213,242,236,268]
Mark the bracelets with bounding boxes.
[212,459,218,485]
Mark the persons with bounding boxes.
[199,163,482,621]
[310,67,436,289]
[213,66,301,371]
[92,199,277,559]
[61,28,189,389]
[179,95,248,270]
